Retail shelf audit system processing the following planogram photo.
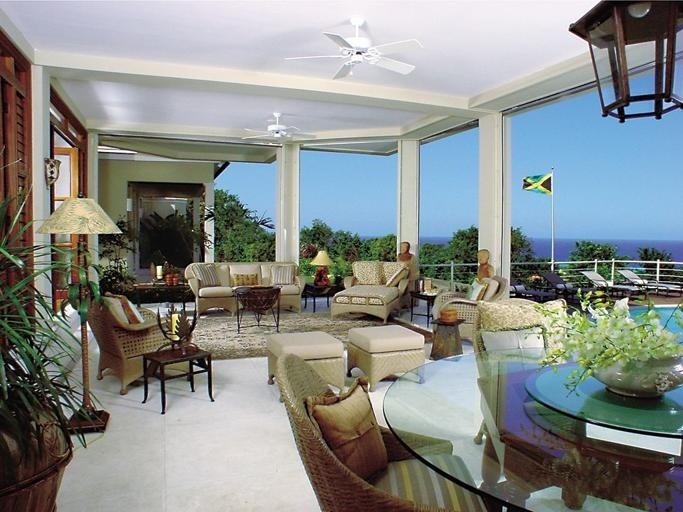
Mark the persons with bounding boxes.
[397,242,414,261]
[477,249,494,278]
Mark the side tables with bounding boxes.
[409,291,437,328]
[429,318,466,359]
[142,346,214,414]
[301,283,336,313]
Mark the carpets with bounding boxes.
[188,313,432,362]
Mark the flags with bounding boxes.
[523,173,553,196]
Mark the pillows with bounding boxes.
[352,260,408,287]
[304,374,391,489]
[479,300,565,355]
[468,277,499,301]
[191,262,294,286]
[102,291,144,327]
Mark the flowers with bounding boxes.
[523,287,683,398]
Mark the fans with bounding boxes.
[284,17,423,79]
[241,113,316,142]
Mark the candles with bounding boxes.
[156,266,162,279]
[171,313,181,340]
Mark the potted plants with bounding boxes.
[162,261,174,285]
[0,147,98,512]
[172,266,182,285]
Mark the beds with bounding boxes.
[331,263,409,324]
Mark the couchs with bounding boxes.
[184,261,300,319]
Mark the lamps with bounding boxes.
[569,0,683,123]
[308,251,335,289]
[35,197,123,435]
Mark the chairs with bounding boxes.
[510,269,683,301]
[84,294,190,395]
[474,297,571,483]
[431,276,507,343]
[276,353,487,512]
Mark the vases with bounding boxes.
[591,357,683,397]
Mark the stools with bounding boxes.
[347,324,426,391]
[266,329,347,396]
